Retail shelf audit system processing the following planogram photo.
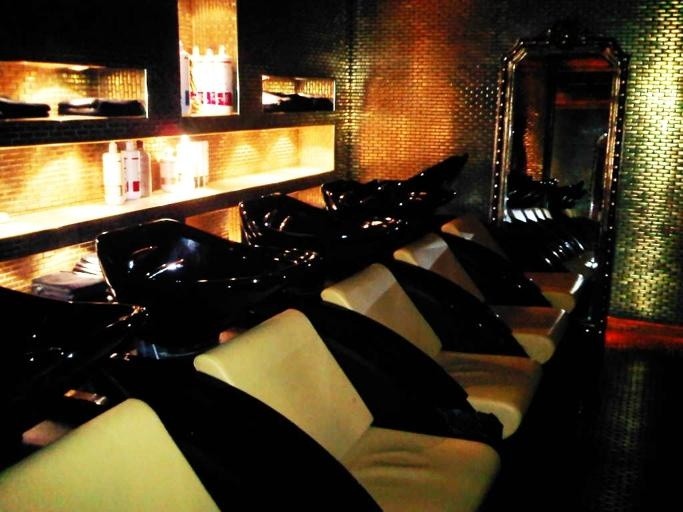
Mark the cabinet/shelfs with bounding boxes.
[0,111,338,263]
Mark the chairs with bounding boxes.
[0,155,585,512]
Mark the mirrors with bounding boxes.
[486,36,629,336]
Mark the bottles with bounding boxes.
[177,39,234,116]
[157,146,178,193]
[101,140,151,205]
